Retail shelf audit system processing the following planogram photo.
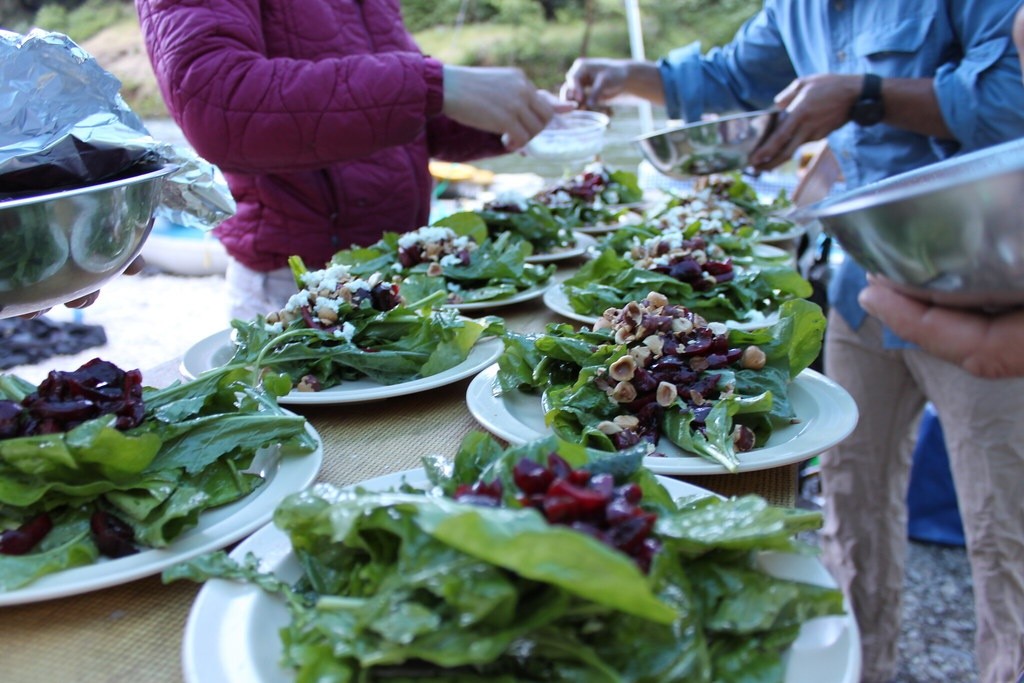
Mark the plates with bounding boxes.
[181,464,861,683]
[0,404,325,609]
[467,362,862,475]
[342,181,815,333]
[177,316,507,405]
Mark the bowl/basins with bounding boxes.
[0,155,193,322]
[523,111,609,164]
[808,137,1024,313]
[629,107,784,182]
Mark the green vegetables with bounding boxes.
[1,170,844,683]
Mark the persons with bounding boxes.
[132,0,574,348]
[557,1,1024,681]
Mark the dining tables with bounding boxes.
[0,182,799,683]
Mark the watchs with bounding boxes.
[848,74,886,127]
